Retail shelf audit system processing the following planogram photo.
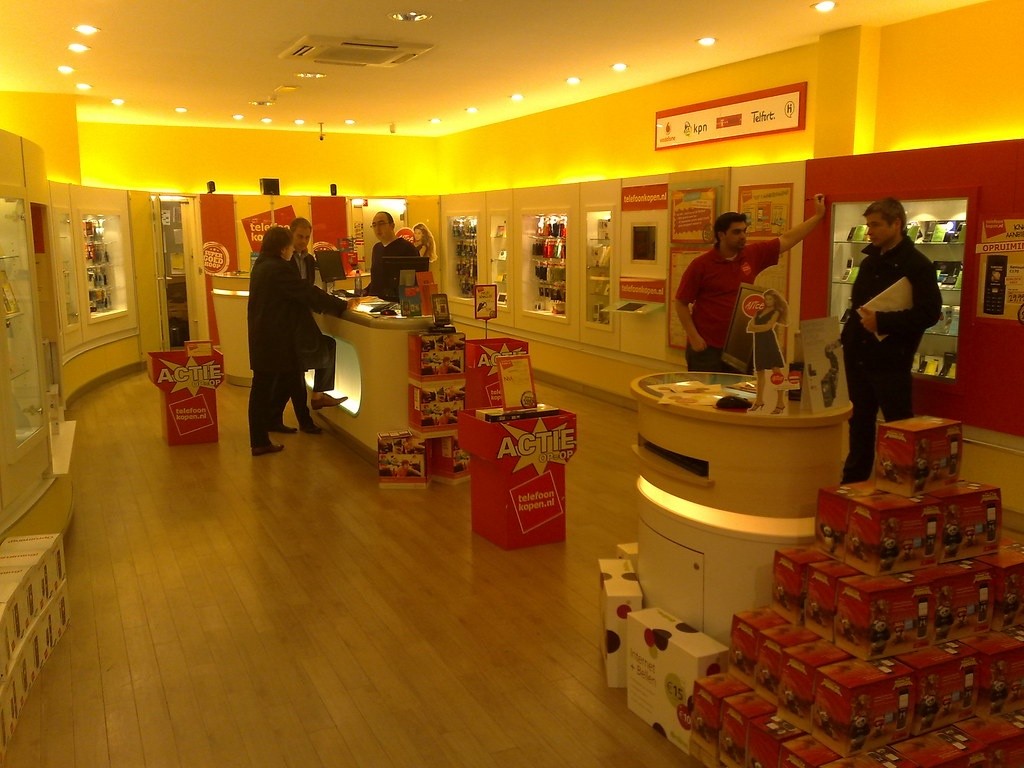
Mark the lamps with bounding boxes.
[330,184,337,195]
[207,181,215,194]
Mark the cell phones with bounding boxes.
[984,255,1007,315]
[846,227,856,241]
[841,298,851,322]
[917,222,958,377]
[841,257,854,281]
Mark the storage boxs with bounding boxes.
[465,337,529,409]
[433,437,470,486]
[408,330,466,382]
[592,480,1024,768]
[875,416,963,498]
[408,377,466,439]
[377,429,432,490]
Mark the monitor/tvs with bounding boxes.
[633,225,656,261]
[378,257,429,303]
[721,281,772,375]
[314,250,346,297]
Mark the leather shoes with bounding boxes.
[252,443,284,456]
[311,393,348,410]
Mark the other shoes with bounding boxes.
[301,424,322,433]
[269,423,298,433]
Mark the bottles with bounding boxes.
[355,270,362,297]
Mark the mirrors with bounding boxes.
[364,197,408,272]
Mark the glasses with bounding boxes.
[370,222,389,228]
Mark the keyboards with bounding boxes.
[370,302,395,313]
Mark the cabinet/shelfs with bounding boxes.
[582,204,617,334]
[830,197,967,380]
[486,209,512,315]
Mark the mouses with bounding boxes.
[716,395,753,409]
[380,309,397,316]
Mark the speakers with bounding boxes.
[259,179,279,195]
[207,181,215,193]
[330,184,336,196]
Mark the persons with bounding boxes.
[841,199,944,484]
[747,289,788,415]
[364,211,420,302]
[269,218,321,433]
[421,335,466,374]
[674,194,826,372]
[414,225,436,261]
[379,436,425,478]
[247,226,360,457]
[420,387,464,427]
